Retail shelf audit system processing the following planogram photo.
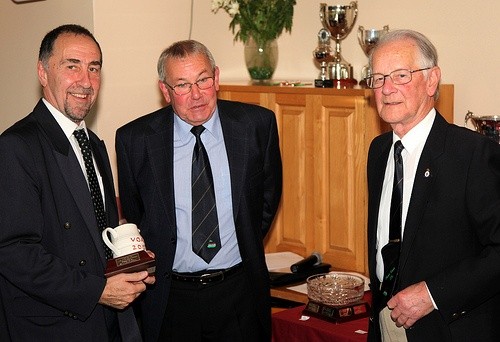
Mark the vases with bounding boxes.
[244,38,278,86]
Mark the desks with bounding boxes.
[269,282,371,342]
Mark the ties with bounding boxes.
[381,141,404,301]
[73,128,113,261]
[190,125,222,265]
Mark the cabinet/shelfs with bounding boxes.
[216,82,454,281]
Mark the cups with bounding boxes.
[102,223,147,259]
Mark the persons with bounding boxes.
[114,40,283,342]
[365,32,500,342]
[0,25,157,342]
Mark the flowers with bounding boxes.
[211,0,296,78]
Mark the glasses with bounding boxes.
[363,66,432,89]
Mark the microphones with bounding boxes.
[290,252,322,272]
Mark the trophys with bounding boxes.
[358,24,388,87]
[318,1,358,85]
[313,29,334,88]
[463,109,499,141]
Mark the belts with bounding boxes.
[171,262,244,286]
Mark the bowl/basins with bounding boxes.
[307,273,365,305]
